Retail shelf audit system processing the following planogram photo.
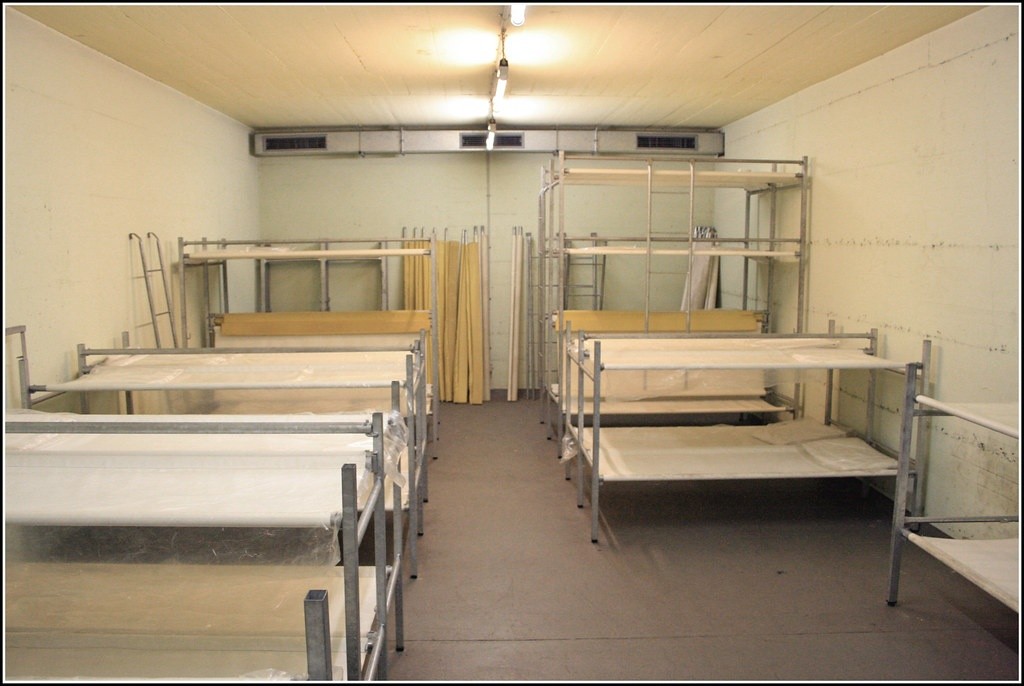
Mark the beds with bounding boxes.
[540,150,1019,613]
[5,233,440,681]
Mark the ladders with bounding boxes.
[128,231,179,349]
[523,233,607,403]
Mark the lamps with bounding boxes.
[485,31,508,150]
[510,4,526,27]
